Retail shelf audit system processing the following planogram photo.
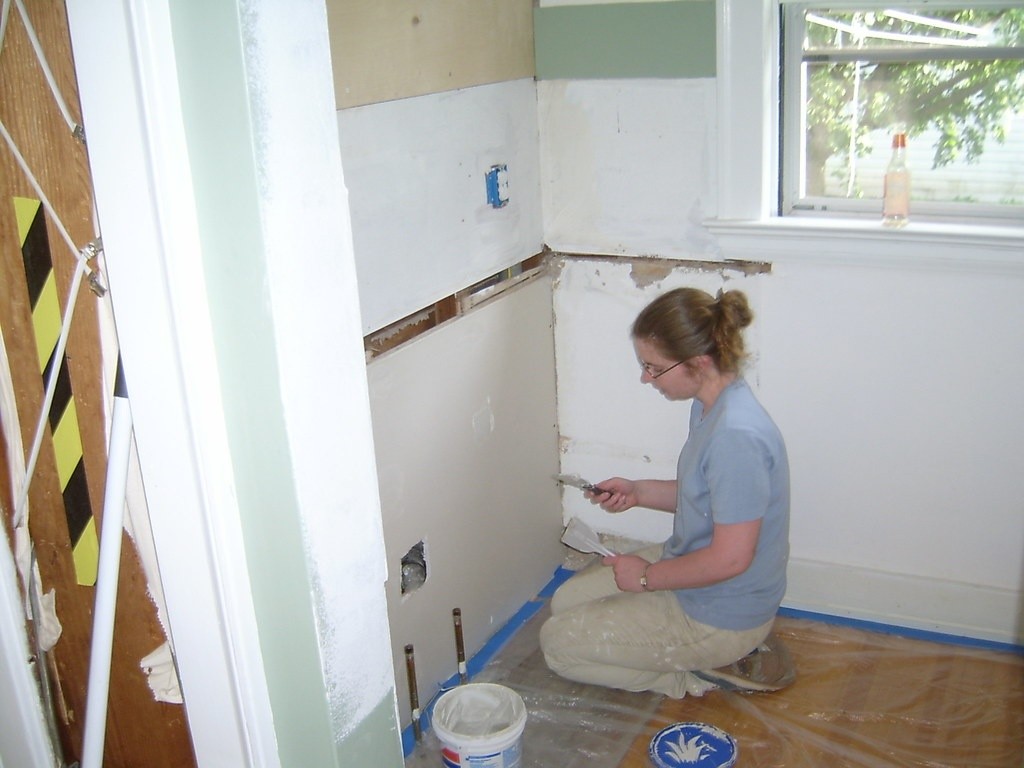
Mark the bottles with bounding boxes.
[882,133,912,231]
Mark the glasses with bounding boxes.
[638,356,693,381]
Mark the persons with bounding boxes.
[539,288,797,699]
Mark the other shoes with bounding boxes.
[691,632,796,693]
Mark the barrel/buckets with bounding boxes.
[431,683,528,768]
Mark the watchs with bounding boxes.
[640,564,653,592]
